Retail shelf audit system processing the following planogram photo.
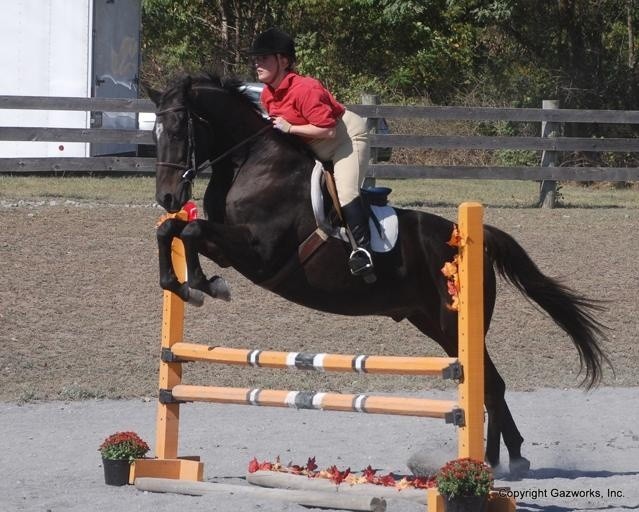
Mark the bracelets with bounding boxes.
[287,124,292,133]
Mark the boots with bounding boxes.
[340,197,371,271]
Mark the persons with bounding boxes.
[237,28,381,287]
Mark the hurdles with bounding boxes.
[127,200,517,508]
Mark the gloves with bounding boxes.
[273,117,291,133]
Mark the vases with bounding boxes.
[101,457,131,486]
[444,494,486,512]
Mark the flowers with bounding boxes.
[434,459,494,499]
[97,432,149,458]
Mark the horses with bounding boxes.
[138,63,616,476]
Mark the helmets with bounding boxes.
[239,31,295,56]
